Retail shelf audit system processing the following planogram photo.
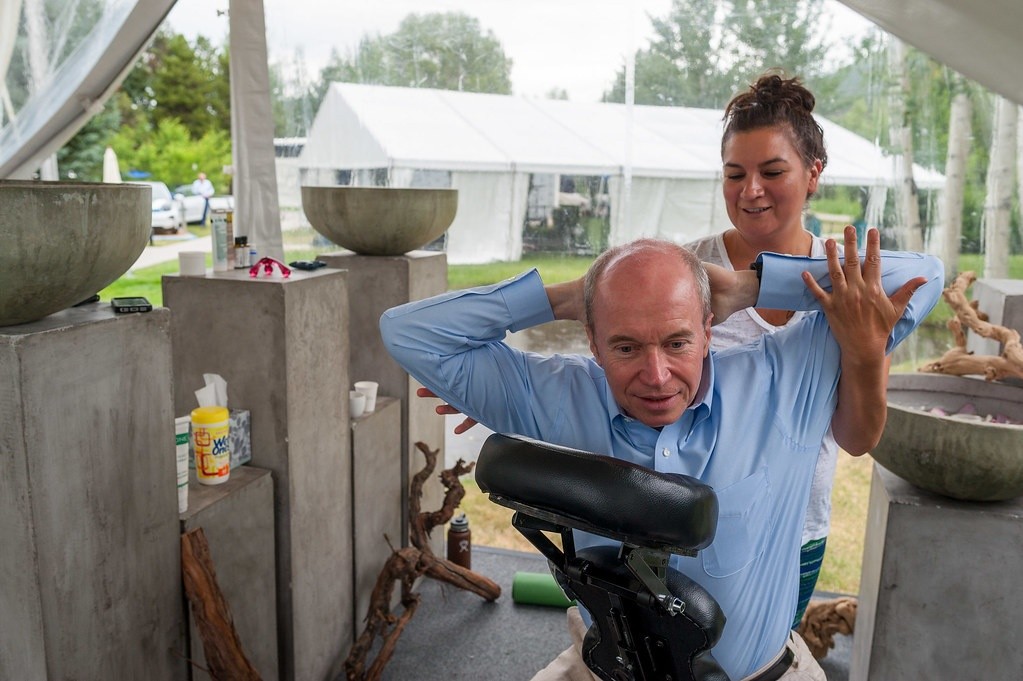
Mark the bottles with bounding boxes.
[233,237,252,269]
[191,405,231,485]
[446,514,471,572]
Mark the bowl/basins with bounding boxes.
[0,179,152,327]
[301,185,460,257]
[866,373,1023,501]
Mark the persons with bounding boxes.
[192,172,215,225]
[417,73,927,627]
[379,241,945,681]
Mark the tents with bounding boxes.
[296,77,942,266]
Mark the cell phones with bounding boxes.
[112,295,153,314]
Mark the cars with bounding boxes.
[121,170,208,235]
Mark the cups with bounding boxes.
[178,251,206,277]
[353,380,378,412]
[348,390,366,418]
[512,572,577,607]
